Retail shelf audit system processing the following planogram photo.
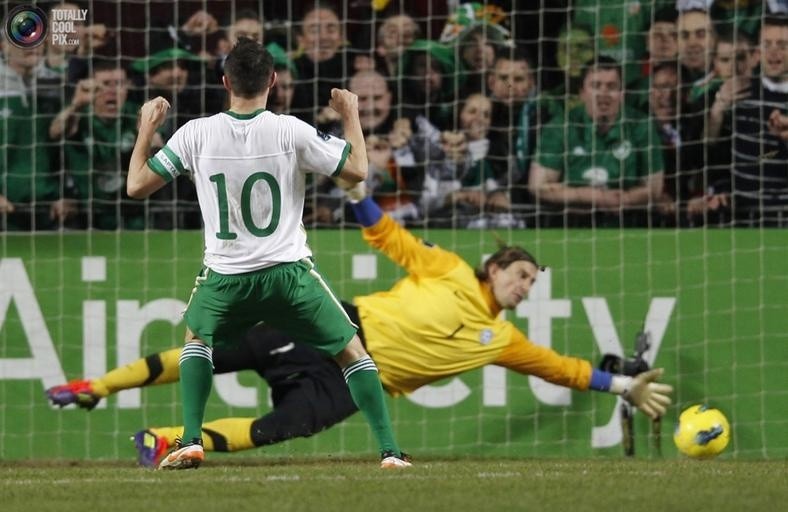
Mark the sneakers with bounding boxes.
[380,450,413,468]
[157,438,205,470]
[134,429,168,467]
[45,381,100,411]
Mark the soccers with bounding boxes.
[674,404,730,458]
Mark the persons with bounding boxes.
[44,172,674,468]
[123,36,413,471]
[0,0,786,232]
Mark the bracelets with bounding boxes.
[588,370,612,392]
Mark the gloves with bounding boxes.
[612,367,673,420]
